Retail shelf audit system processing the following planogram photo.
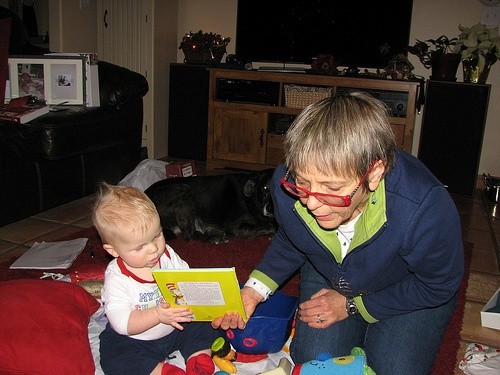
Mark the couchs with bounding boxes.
[0,61,149,227]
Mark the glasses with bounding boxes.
[280,161,376,207]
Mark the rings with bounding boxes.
[317,315,322,322]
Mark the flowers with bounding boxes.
[458,21,500,69]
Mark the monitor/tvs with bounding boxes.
[235,0,413,69]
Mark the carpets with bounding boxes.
[0,227,473,375]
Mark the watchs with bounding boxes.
[345,296,359,317]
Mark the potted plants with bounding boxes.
[409,34,462,80]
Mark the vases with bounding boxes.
[464,54,495,84]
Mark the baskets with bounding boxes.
[284,85,332,109]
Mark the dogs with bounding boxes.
[143,167,279,245]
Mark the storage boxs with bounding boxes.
[165,158,197,178]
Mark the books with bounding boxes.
[0,103,49,124]
[151,266,246,323]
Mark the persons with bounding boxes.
[21,73,33,92]
[212,91,464,375]
[93,181,218,375]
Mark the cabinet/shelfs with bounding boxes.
[206,68,415,172]
